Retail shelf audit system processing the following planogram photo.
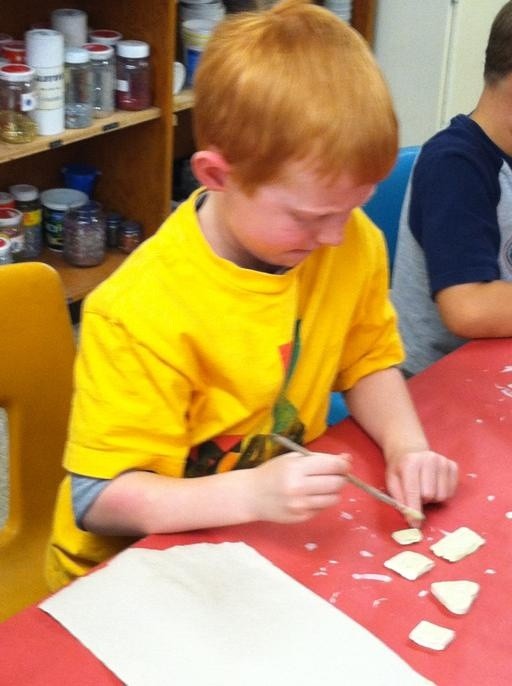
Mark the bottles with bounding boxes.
[0,9,152,151]
[1,164,144,269]
[174,0,353,98]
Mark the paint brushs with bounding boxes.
[273,434,426,521]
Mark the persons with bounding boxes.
[386,1,512,390]
[41,1,462,596]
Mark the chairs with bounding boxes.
[0,261,79,625]
[324,140,429,431]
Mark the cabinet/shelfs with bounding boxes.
[0,0,379,306]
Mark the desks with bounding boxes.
[2,335,512,685]
